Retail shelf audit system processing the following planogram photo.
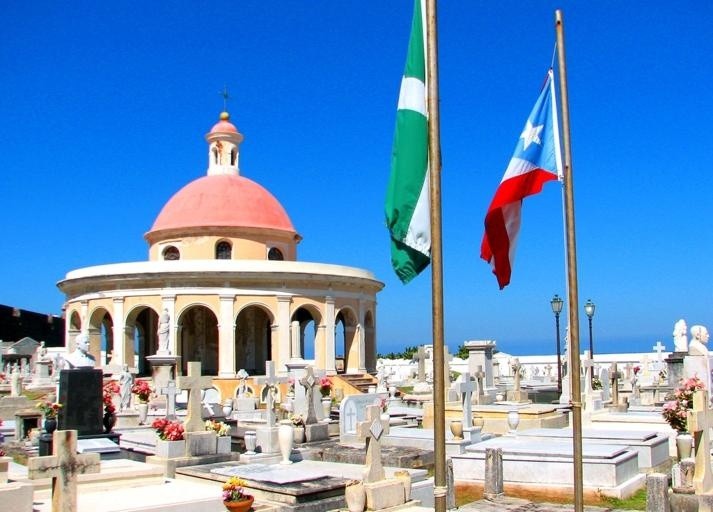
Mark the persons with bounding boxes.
[66,333,96,370]
[119,364,133,408]
[688,325,709,356]
[157,307,170,351]
[36,341,48,357]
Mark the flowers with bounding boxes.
[658,373,709,431]
[205,418,232,437]
[318,376,334,396]
[152,417,184,441]
[102,381,121,413]
[132,380,152,401]
[222,476,249,502]
[36,400,65,419]
[290,413,305,427]
[287,378,295,392]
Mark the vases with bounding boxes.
[224,495,254,512]
[44,416,57,434]
[293,425,305,443]
[104,412,117,434]
[321,395,332,421]
[676,432,693,461]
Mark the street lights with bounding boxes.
[551,294,564,398]
[584,297,595,387]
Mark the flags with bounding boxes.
[384,0,431,286]
[480,69,563,290]
